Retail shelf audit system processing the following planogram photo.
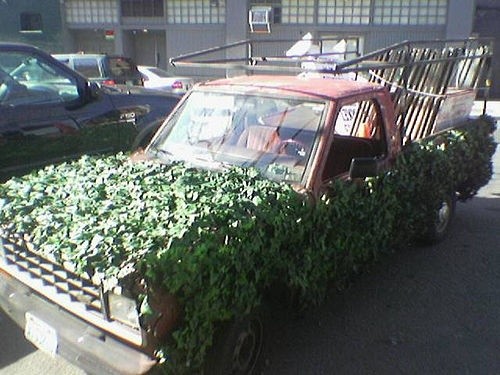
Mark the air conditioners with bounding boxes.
[249,5,274,35]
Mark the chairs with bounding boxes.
[235,123,285,153]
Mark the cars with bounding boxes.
[136,64,194,95]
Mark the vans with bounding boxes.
[0,54,144,95]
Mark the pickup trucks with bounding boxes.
[0,41,182,186]
[1,34,499,375]
